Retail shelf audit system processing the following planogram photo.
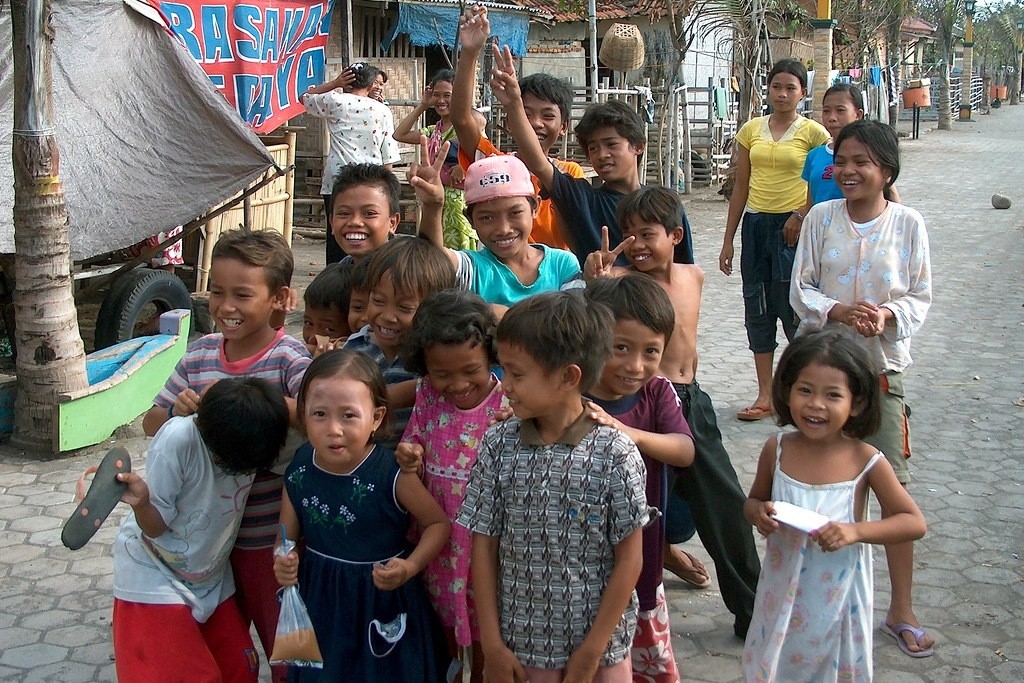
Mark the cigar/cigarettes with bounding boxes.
[460,10,486,29]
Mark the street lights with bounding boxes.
[1009,22,1023,104]
[958,1,977,123]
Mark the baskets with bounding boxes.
[598,23,644,72]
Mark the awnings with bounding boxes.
[0,0,296,298]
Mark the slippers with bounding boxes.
[881,618,933,658]
[663,550,712,588]
[737,406,776,419]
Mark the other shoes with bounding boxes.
[62,447,131,550]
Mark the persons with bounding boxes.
[742,327,926,682]
[719,58,900,421]
[60,4,762,683]
[789,119,936,657]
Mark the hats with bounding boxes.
[464,155,535,205]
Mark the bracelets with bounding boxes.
[168,403,175,418]
[792,210,804,221]
